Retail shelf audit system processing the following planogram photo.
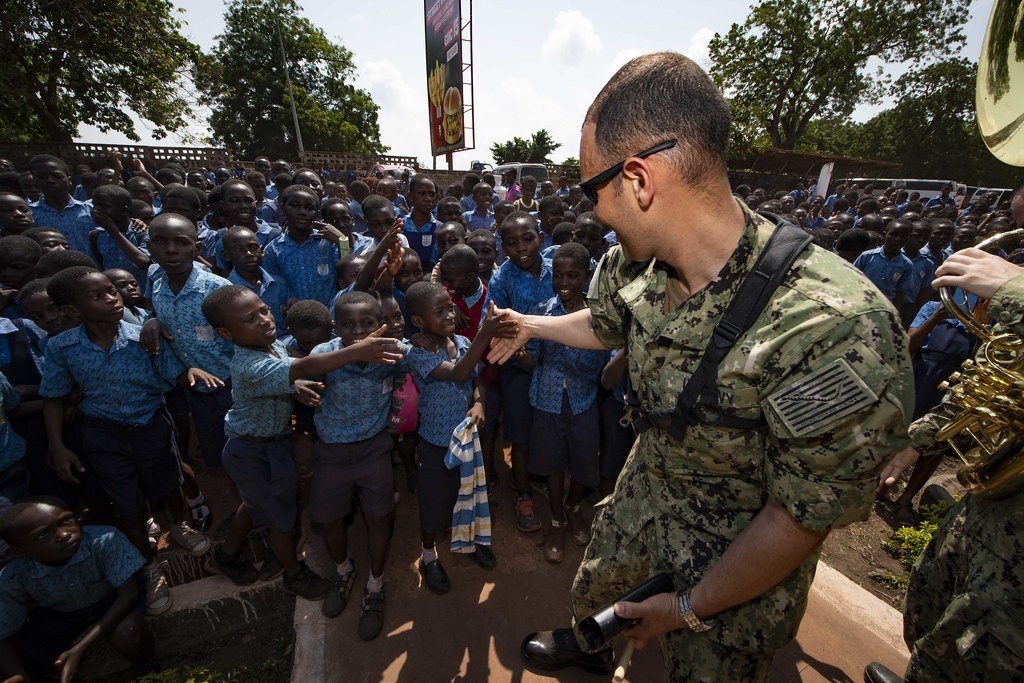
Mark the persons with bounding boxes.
[483,47,915,681]
[858,250,1024,681]
[733,161,1024,523]
[2,146,629,683]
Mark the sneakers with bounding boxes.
[170,522,211,556]
[322,557,357,618]
[284,562,332,601]
[357,582,385,640]
[544,511,564,563]
[514,495,541,532]
[471,543,498,569]
[564,497,591,545]
[206,541,261,586]
[419,555,451,593]
[142,559,173,615]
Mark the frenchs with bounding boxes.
[932,0,1024,496]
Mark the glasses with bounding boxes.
[577,138,676,205]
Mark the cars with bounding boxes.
[358,161,422,205]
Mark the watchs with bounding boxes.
[677,586,721,634]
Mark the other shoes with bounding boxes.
[191,497,215,532]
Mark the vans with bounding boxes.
[490,160,551,201]
